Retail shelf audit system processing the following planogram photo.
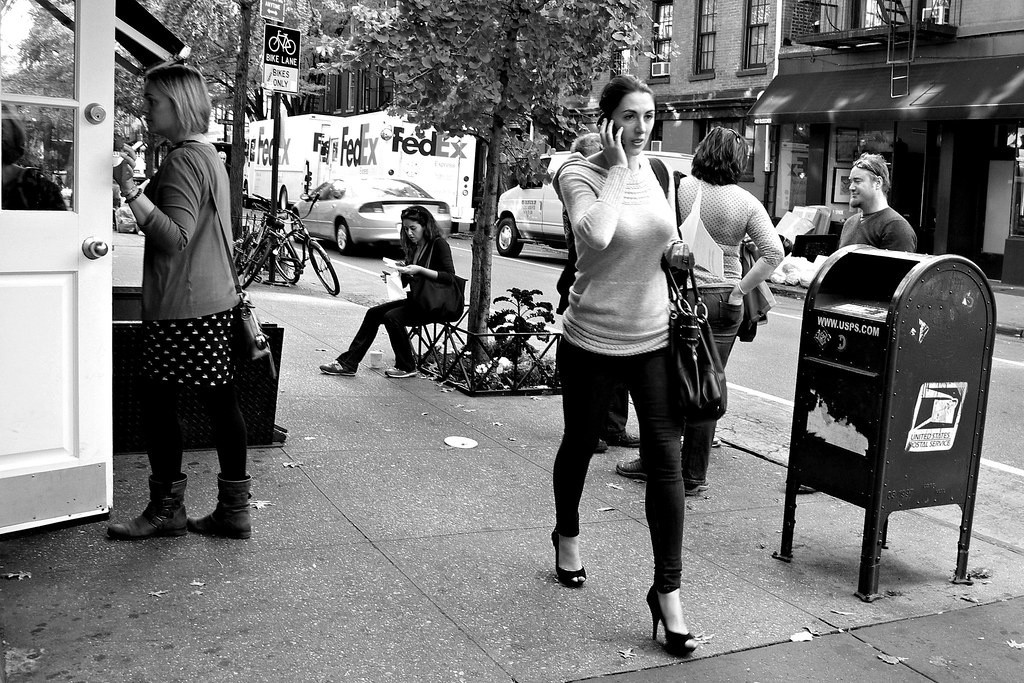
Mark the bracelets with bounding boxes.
[125,188,142,203]
[120,182,136,197]
[737,282,747,295]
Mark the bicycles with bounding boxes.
[233,192,341,297]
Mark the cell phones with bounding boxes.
[596,114,626,149]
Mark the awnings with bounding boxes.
[747,53,1024,125]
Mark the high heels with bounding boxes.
[550,527,586,588]
[647,583,697,656]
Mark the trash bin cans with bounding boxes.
[778,243,997,601]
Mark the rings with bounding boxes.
[683,259,688,265]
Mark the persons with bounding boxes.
[1,103,68,211]
[558,133,638,451]
[553,75,699,656]
[108,63,254,539]
[616,127,785,496]
[320,205,456,379]
[839,152,918,253]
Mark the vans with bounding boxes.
[494,151,696,257]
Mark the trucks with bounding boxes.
[243,110,477,233]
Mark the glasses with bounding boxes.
[725,128,741,144]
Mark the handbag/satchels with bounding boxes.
[661,254,728,421]
[233,288,276,382]
[419,275,463,322]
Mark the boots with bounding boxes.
[185,473,252,539]
[107,472,188,539]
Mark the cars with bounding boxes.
[291,175,451,255]
[112,156,146,183]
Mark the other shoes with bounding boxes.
[685,480,710,496]
[616,458,647,481]
[712,435,721,447]
[595,433,640,451]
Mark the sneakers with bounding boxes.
[319,360,356,376]
[385,367,417,378]
